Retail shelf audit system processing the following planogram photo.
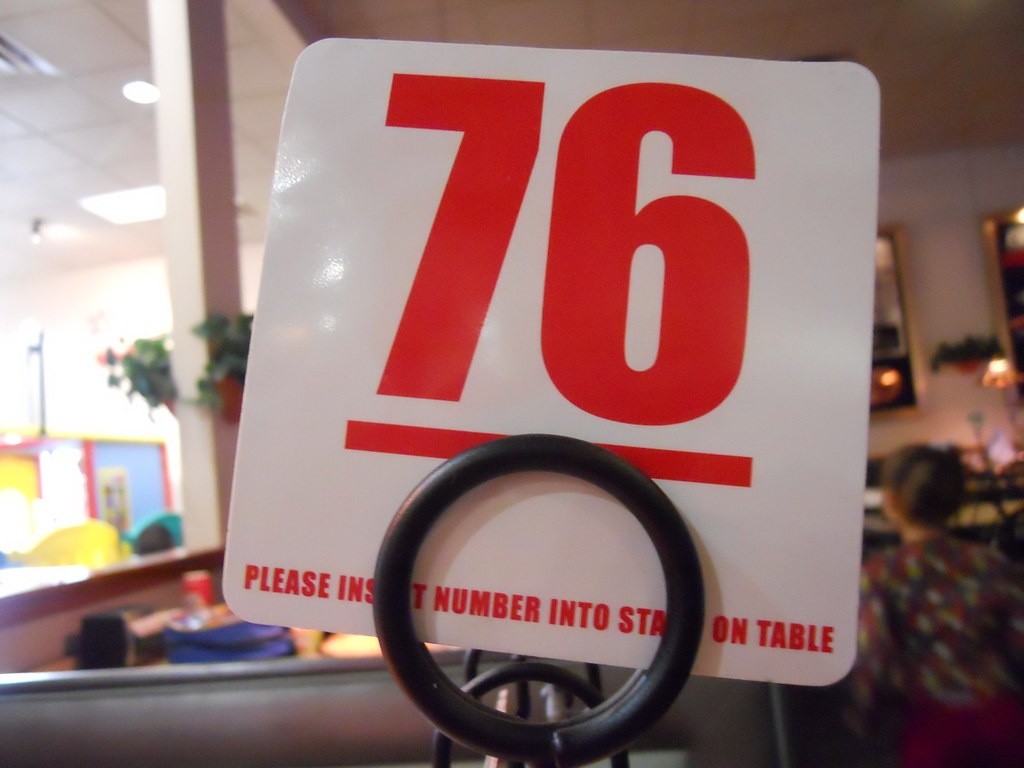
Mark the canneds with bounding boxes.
[179,569,214,612]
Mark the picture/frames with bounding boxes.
[869,223,922,421]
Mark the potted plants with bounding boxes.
[107,338,175,416]
[190,312,255,422]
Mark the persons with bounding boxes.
[849,443,1024,768]
[65,611,128,669]
